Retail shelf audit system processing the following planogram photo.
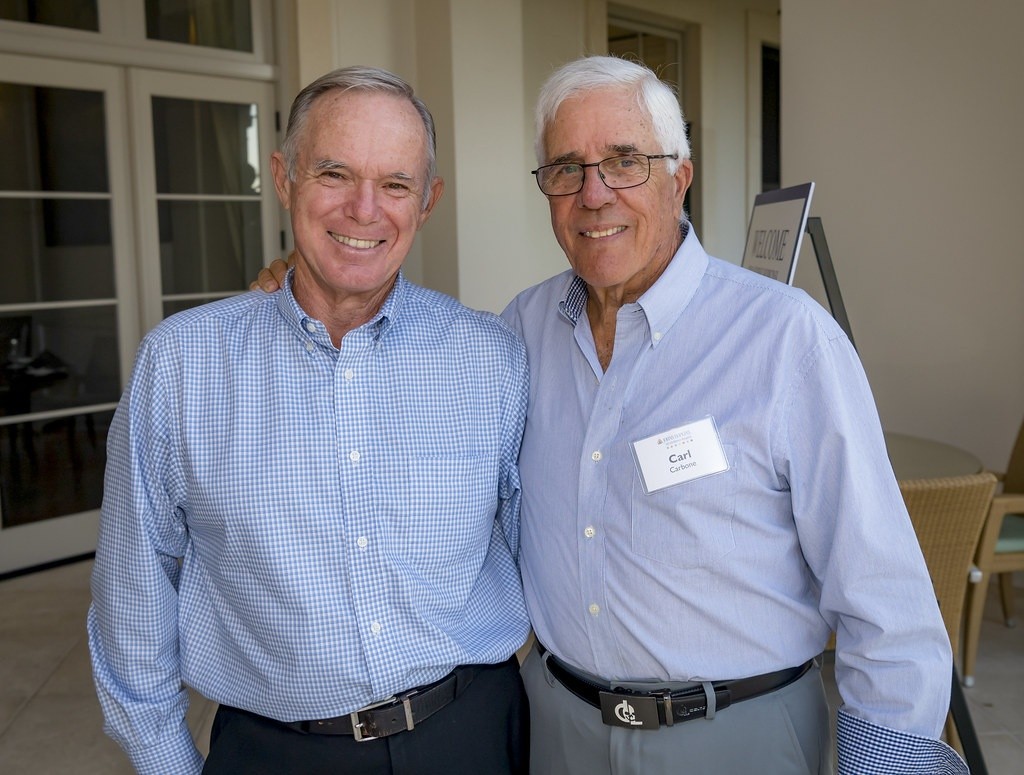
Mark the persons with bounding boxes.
[84,64,536,775]
[246,56,973,775]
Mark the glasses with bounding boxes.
[531,154,678,196]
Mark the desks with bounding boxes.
[882,428,983,478]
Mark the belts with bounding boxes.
[534,633,814,730]
[284,665,485,742]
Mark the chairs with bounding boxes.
[0,315,97,483]
[822,420,1024,755]
